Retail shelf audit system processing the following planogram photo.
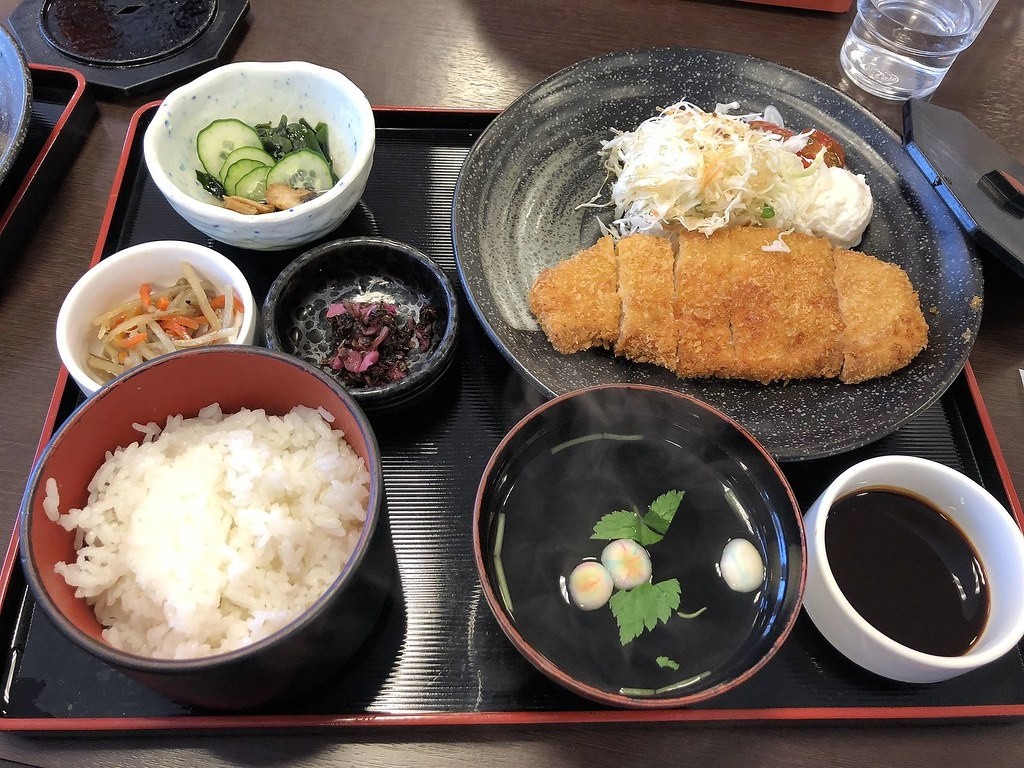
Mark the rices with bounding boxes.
[42,400,369,662]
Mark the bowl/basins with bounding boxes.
[142,59,376,251]
[55,238,258,404]
[262,237,457,415]
[795,453,1024,684]
[15,345,385,708]
[475,384,808,709]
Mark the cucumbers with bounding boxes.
[195,116,334,200]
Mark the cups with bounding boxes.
[838,0,999,102]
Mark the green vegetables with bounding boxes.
[608,578,682,646]
[589,488,685,545]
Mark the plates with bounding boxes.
[449,47,985,465]
[0,24,32,189]
